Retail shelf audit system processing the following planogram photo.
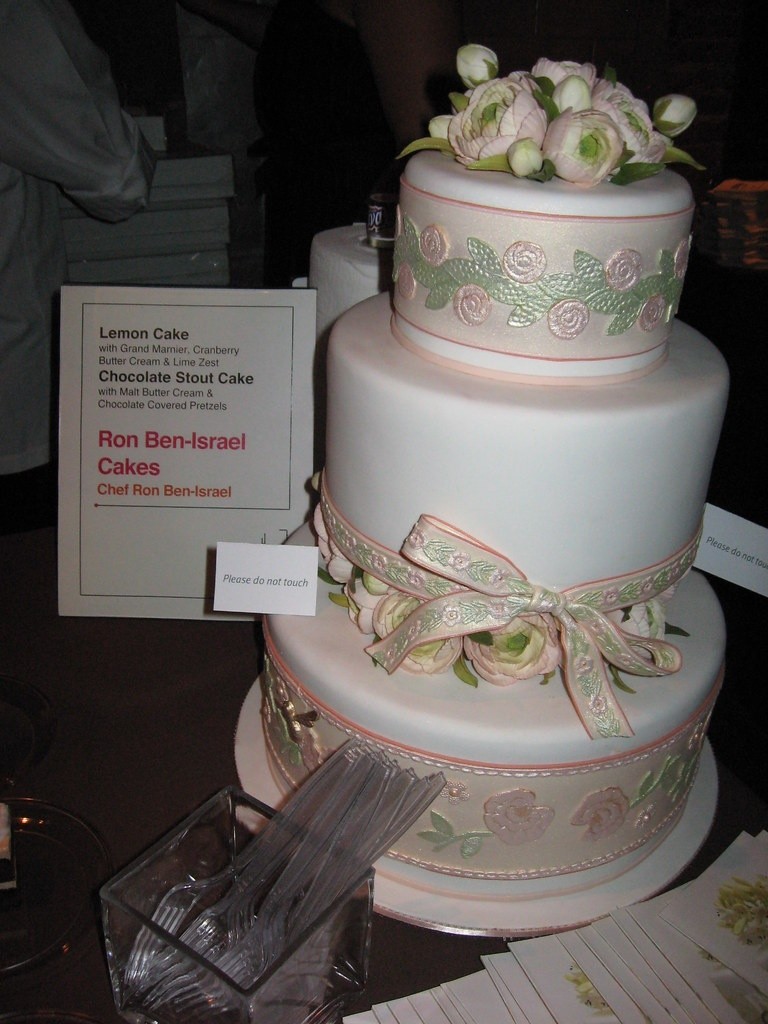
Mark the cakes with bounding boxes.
[263,153,731,898]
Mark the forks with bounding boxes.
[125,740,446,1024]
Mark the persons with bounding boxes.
[1,1,154,537]
[177,1,457,293]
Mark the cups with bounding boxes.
[368,194,398,249]
[99,783,375,1024]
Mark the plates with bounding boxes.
[0,799,113,998]
[0,676,55,790]
[0,1008,106,1024]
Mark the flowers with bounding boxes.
[606,585,690,695]
[394,43,708,187]
[328,566,388,635]
[372,591,480,688]
[463,612,562,687]
[313,503,354,586]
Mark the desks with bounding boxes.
[0,496,768,1024]
